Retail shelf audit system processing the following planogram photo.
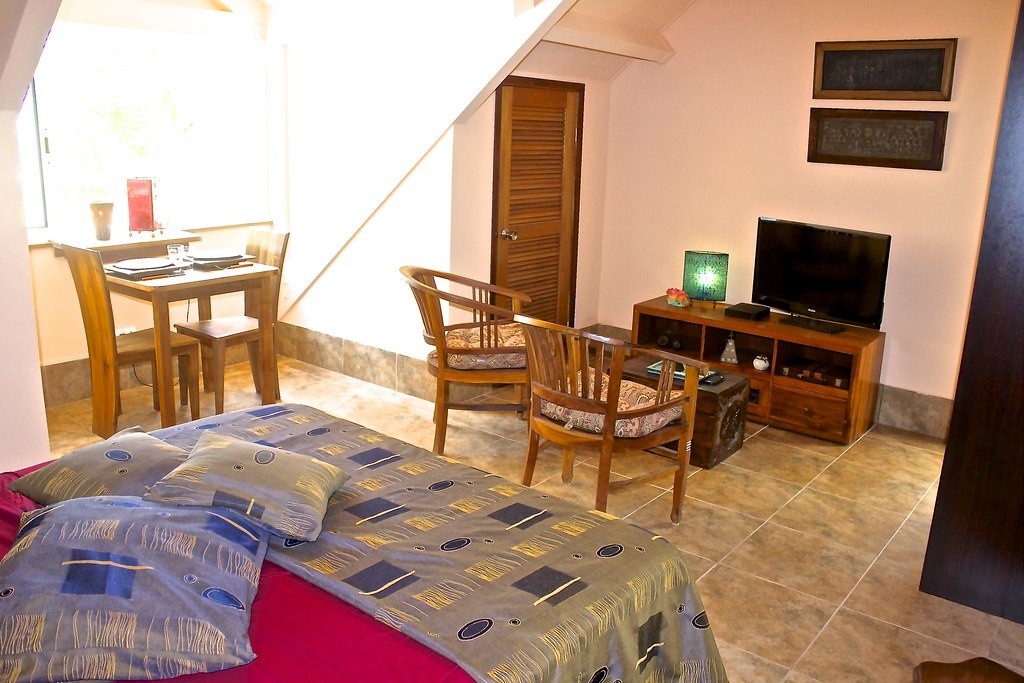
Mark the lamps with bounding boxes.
[682,250,729,307]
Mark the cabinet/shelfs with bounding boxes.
[632,294,886,446]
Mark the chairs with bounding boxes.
[173,229,291,417]
[397,266,536,456]
[513,312,710,526]
[59,244,201,442]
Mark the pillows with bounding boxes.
[0,496,270,681]
[140,429,352,544]
[5,424,190,505]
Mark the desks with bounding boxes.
[609,355,753,469]
[99,256,281,431]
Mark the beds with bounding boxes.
[0,398,730,683]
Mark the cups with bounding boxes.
[166,243,185,263]
[89,201,114,241]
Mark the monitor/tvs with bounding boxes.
[751,217,891,334]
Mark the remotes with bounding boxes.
[705,375,724,385]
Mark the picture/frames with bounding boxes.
[812,38,959,101]
[807,107,949,172]
